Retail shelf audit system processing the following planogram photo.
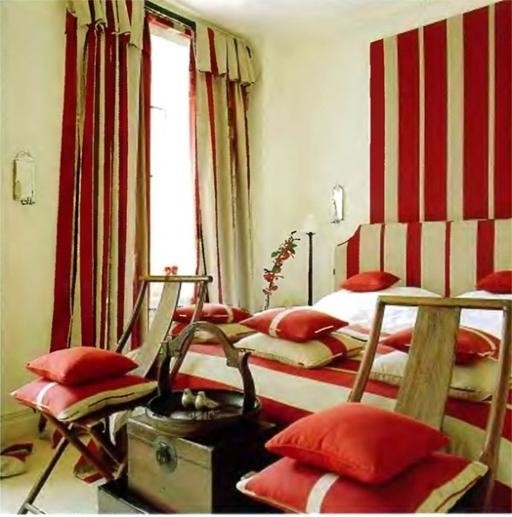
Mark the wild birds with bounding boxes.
[181,388,196,416]
[194,390,221,417]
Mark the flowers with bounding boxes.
[260,230,301,309]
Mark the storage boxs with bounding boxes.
[125,412,276,514]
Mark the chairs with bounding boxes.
[15,276,215,514]
[235,295,512,512]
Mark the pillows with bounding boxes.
[236,400,489,512]
[10,345,158,421]
[173,304,256,344]
[338,271,400,292]
[474,271,512,295]
[234,307,364,364]
[455,291,511,339]
[313,288,441,342]
[369,325,501,400]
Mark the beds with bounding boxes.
[170,219,512,513]
